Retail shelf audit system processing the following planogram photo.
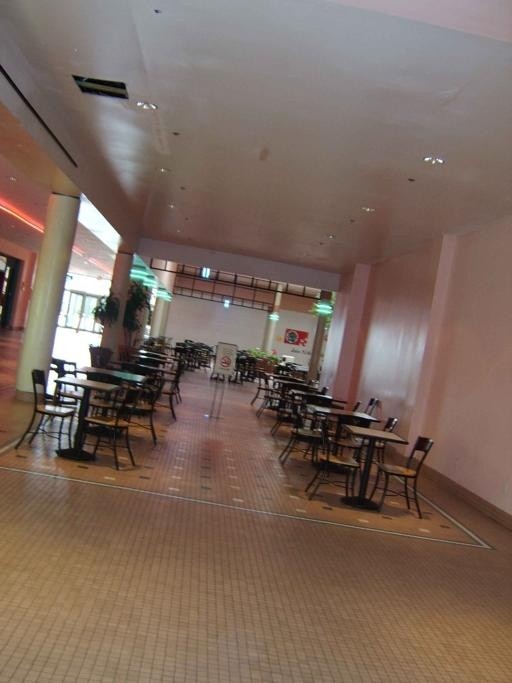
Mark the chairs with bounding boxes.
[173,337,266,385]
[16,337,216,475]
[250,362,432,520]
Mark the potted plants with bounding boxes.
[118,280,152,362]
[87,287,120,367]
